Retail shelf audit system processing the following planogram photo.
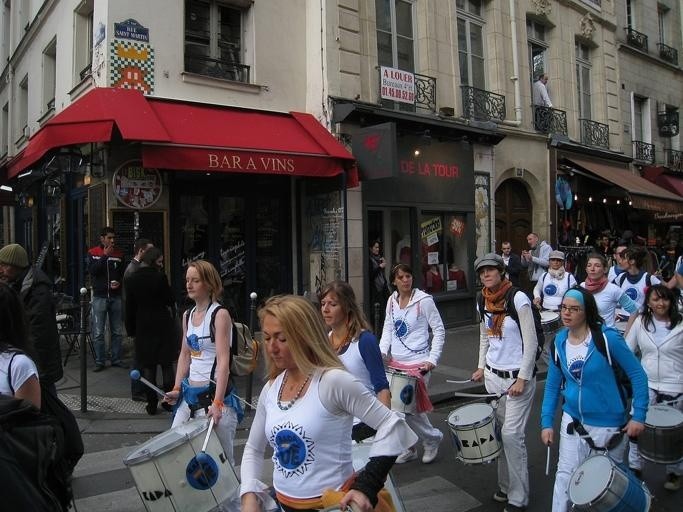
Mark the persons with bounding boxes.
[120,233,445,510]
[472,234,682,511]
[532,73,555,112]
[87,227,130,373]
[0,242,84,512]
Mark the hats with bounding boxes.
[0,244,29,268]
[473,253,506,273]
[549,250,565,260]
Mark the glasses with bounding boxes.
[557,305,581,313]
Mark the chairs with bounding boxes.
[57,304,96,361]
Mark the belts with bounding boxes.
[485,364,524,378]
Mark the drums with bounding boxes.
[567,456,652,507]
[353,449,405,507]
[446,402,504,465]
[389,372,420,417]
[541,310,562,336]
[122,415,242,508]
[638,405,682,464]
[614,321,629,335]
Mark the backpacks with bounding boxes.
[478,286,545,360]
[0,351,84,512]
[185,304,260,413]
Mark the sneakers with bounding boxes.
[112,361,130,368]
[92,364,105,371]
[664,472,683,490]
[422,432,443,463]
[395,448,417,464]
[494,490,527,512]
